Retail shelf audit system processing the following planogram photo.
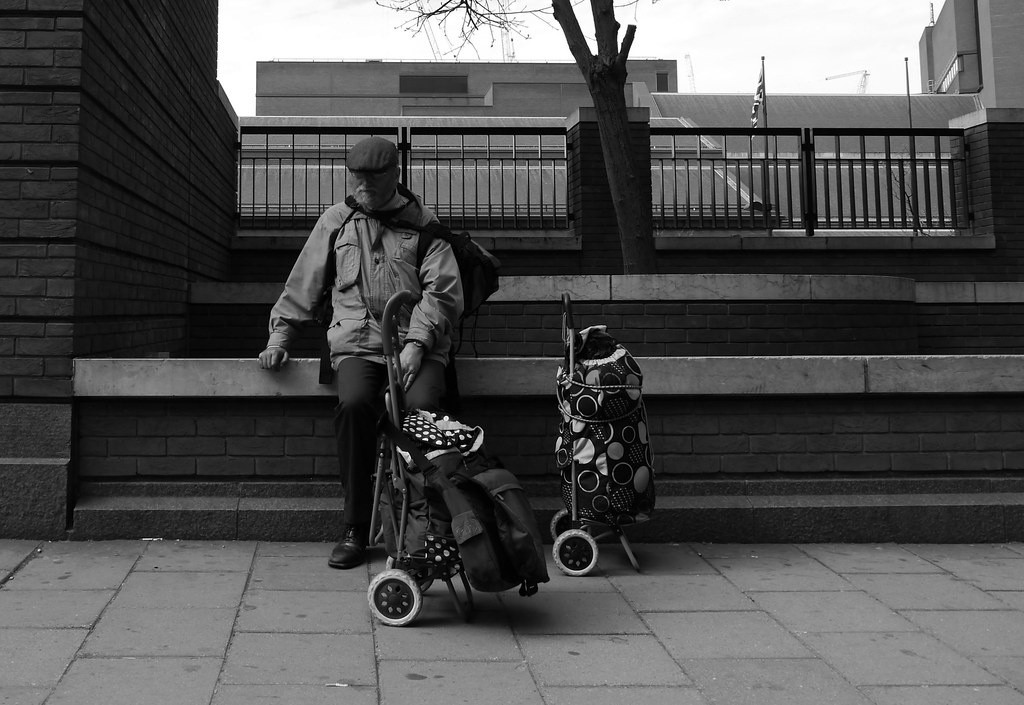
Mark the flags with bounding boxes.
[749,65,765,141]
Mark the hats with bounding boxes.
[345,135,400,173]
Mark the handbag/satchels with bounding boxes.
[557,326,657,532]
[443,468,549,598]
[380,408,492,581]
[449,232,500,316]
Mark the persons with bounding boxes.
[258,135,468,570]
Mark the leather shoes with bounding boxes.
[328,526,366,568]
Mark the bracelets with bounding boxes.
[413,341,422,346]
